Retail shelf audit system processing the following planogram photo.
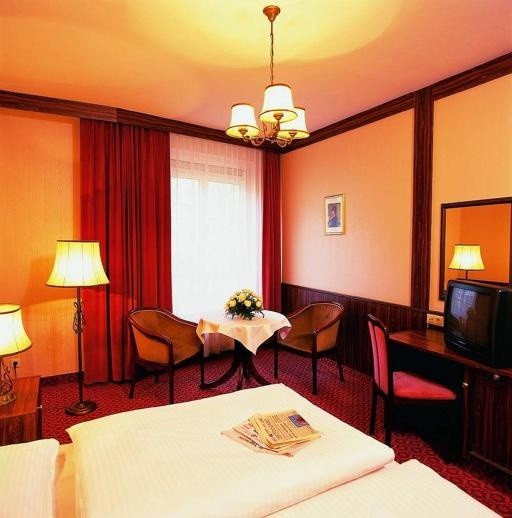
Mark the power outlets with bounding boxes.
[426,313,445,327]
[10,359,20,368]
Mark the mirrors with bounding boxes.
[438,195,512,302]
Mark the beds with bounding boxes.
[0,383,503,518]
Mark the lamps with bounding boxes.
[0,302,32,405]
[448,243,486,280]
[45,239,110,416]
[225,5,310,148]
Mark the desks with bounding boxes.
[388,328,512,477]
[196,310,292,390]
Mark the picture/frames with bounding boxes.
[321,193,345,235]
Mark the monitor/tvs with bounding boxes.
[442,278,512,370]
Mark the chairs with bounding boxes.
[366,314,458,445]
[274,300,344,395]
[128,306,204,404]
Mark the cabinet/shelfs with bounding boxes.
[0,375,43,446]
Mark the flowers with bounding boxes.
[224,289,265,321]
